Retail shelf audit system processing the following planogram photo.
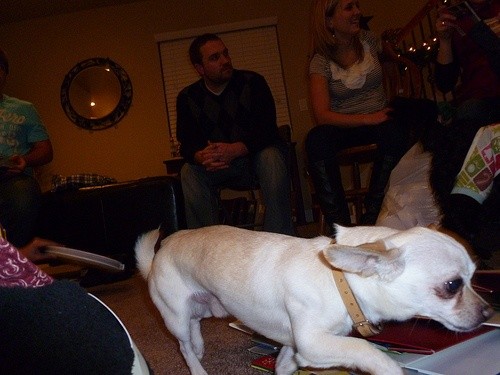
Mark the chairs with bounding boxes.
[318,142,381,238]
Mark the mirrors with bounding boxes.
[60,56,133,132]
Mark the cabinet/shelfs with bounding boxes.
[165,141,307,229]
[63,175,177,283]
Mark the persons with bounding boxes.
[304,0,441,228]
[0,51,54,248]
[0,231,148,375]
[436,0,500,259]
[176,33,294,234]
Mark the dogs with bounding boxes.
[135,222,494,375]
